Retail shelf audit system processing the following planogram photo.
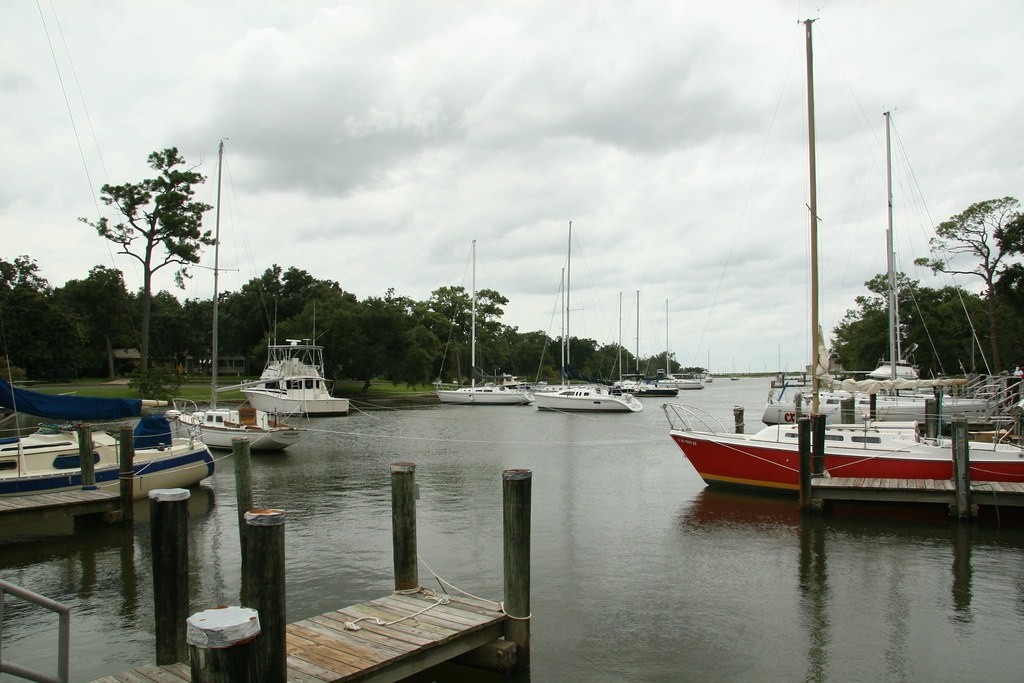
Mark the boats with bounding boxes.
[0,330,217,549]
[239,297,351,419]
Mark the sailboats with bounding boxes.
[662,17,1024,527]
[431,237,537,407]
[163,136,308,454]
[481,109,1024,460]
